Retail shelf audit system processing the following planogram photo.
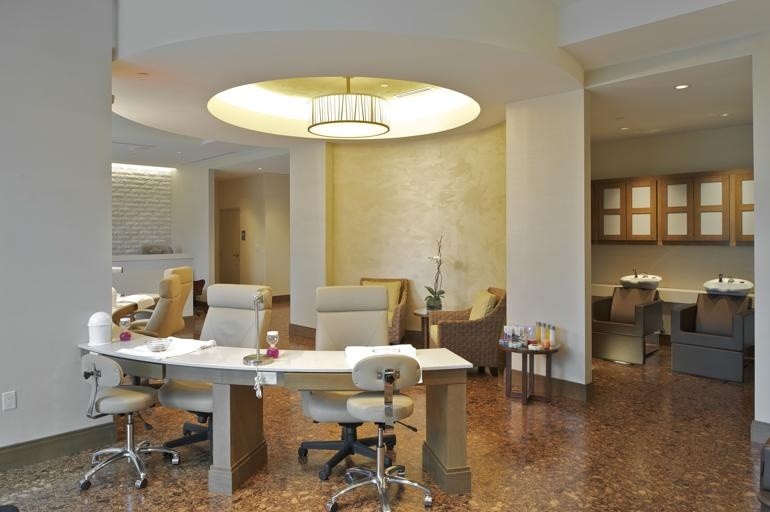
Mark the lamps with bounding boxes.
[308,75,390,139]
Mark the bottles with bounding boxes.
[535,322,557,346]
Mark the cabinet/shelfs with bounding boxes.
[591,168,754,246]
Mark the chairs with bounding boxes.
[429,286,506,377]
[591,287,664,366]
[670,293,755,383]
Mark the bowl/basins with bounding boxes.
[145,339,172,352]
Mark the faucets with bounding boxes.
[719,273,723,282]
[633,268,637,277]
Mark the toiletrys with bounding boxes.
[536,321,555,346]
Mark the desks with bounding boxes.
[78,294,473,494]
[498,340,562,407]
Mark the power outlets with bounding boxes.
[1,391,17,414]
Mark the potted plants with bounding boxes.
[425,234,445,310]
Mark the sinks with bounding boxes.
[621,275,662,289]
[703,278,754,297]
